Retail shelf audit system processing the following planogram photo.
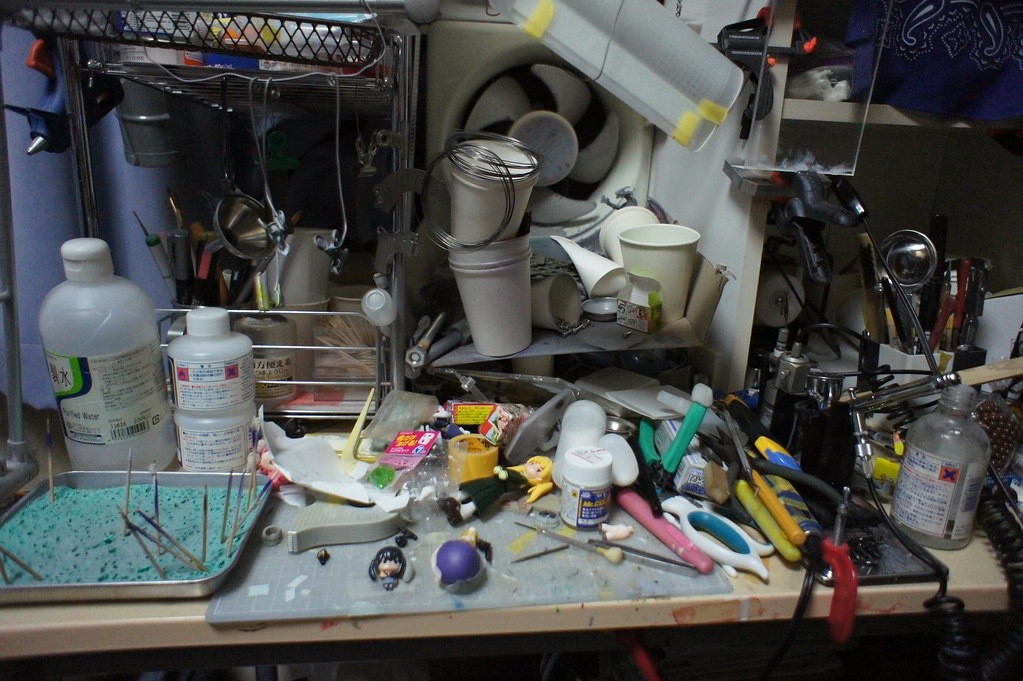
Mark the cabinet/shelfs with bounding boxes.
[0,1,414,481]
[727,0,1023,400]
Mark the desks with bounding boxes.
[0,425,1009,681]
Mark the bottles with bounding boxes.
[233,312,298,408]
[166,307,255,474]
[559,448,615,532]
[37,238,177,472]
[890,384,990,551]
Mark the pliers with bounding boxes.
[638,400,706,495]
[695,425,844,523]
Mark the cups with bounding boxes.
[447,141,540,356]
[530,272,582,333]
[263,228,371,390]
[598,206,701,324]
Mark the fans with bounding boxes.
[425,20,652,254]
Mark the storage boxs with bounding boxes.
[879,342,957,417]
[446,401,533,442]
